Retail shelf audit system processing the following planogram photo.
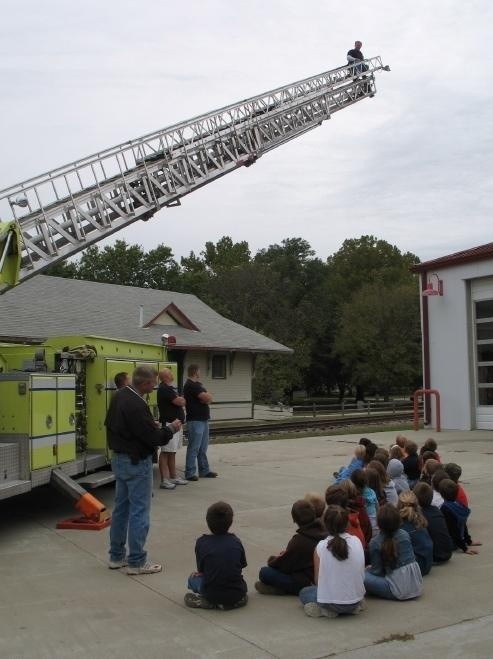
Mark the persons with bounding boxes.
[342,41,365,78]
[156,367,189,490]
[105,364,180,577]
[253,438,484,619]
[180,502,249,609]
[113,372,129,391]
[183,364,219,481]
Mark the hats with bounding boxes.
[386,458,404,479]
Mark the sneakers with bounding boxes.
[159,479,175,489]
[199,471,218,477]
[253,579,281,594]
[301,600,340,619]
[125,562,163,576]
[105,555,129,569]
[183,591,215,610]
[170,477,188,484]
[188,476,198,481]
[216,593,249,610]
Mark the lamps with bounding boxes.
[422,274,443,297]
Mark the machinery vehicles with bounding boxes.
[1,52,392,528]
